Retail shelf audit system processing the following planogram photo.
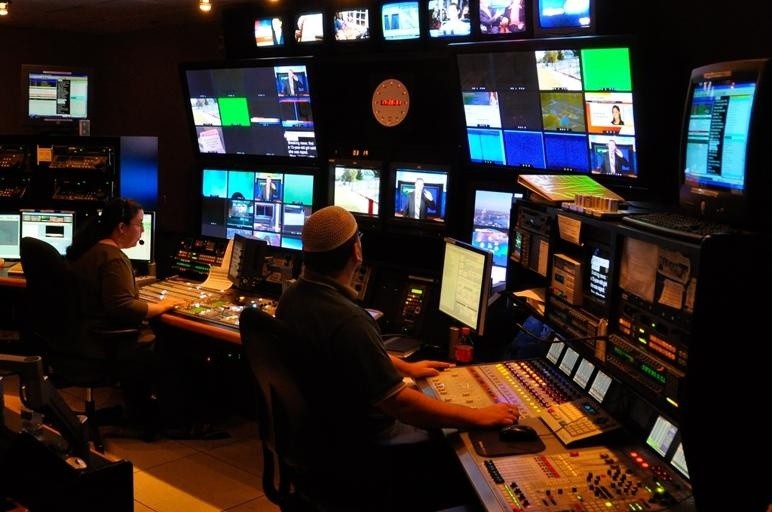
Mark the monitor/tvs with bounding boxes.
[491,265,506,285]
[393,163,453,227]
[327,157,386,224]
[333,8,372,43]
[253,15,287,50]
[470,227,510,268]
[471,189,514,230]
[436,234,493,339]
[198,154,326,254]
[0,213,20,260]
[427,0,474,39]
[179,55,323,166]
[291,11,330,48]
[446,33,643,185]
[96,207,158,265]
[381,1,422,42]
[681,58,771,200]
[17,61,92,120]
[532,0,597,34]
[478,0,530,36]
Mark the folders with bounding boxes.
[517,174,626,203]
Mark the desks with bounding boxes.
[411,341,676,512]
[4,259,284,346]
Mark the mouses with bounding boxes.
[499,423,538,442]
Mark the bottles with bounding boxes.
[454,327,474,365]
[447,327,460,362]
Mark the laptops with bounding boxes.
[7,208,75,277]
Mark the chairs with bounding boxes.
[236,302,334,511]
[19,236,160,452]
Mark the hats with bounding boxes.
[301,206,359,252]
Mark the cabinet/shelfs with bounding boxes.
[504,194,771,512]
[0,133,157,231]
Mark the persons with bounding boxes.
[276,206,520,512]
[600,140,629,174]
[403,178,435,220]
[611,106,624,125]
[284,70,303,97]
[428,0,525,37]
[63,198,183,431]
[261,177,279,202]
[271,18,284,45]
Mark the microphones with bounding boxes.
[137,235,145,247]
